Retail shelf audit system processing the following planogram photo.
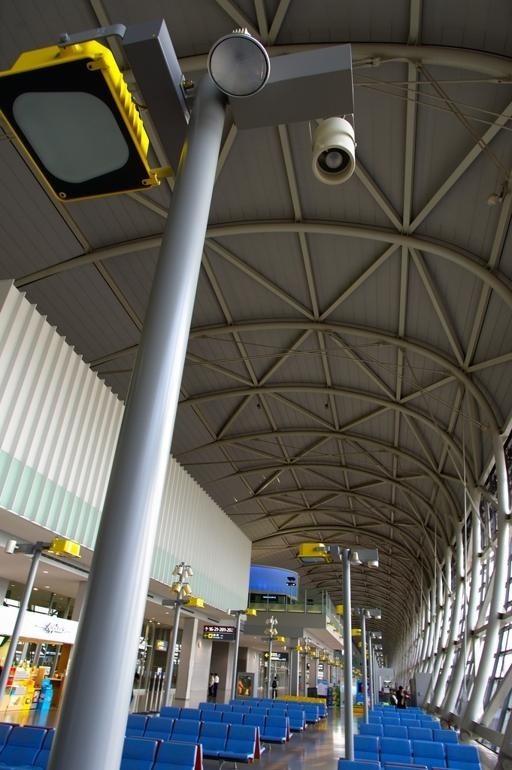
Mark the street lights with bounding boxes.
[162,557,207,710]
[1,21,358,769]
[0,535,81,694]
[228,542,389,761]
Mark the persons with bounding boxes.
[390,686,410,710]
[272,677,278,699]
[208,673,215,696]
[212,673,220,697]
